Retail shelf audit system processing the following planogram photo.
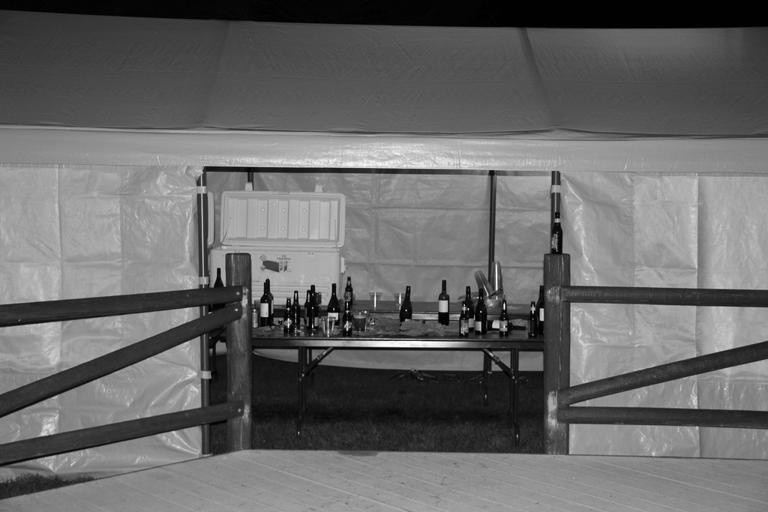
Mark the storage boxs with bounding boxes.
[208,182,348,305]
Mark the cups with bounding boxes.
[321,316,335,337]
[353,316,365,335]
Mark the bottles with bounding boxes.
[399,285,412,327]
[459,286,487,338]
[551,212,563,254]
[260,278,274,327]
[438,280,450,325]
[342,276,352,337]
[326,281,340,336]
[208,268,225,315]
[500,300,509,338]
[528,284,545,340]
[283,291,301,337]
[304,285,319,337]
